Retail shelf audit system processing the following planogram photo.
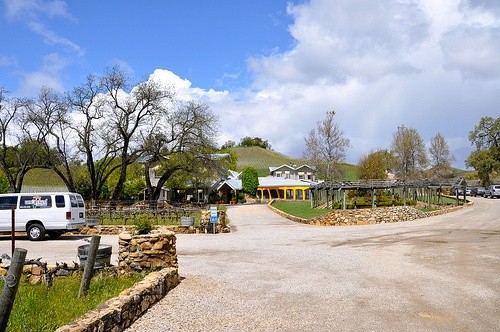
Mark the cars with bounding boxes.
[461,187,473,195]
[470,187,484,197]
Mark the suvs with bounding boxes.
[483,185,500,198]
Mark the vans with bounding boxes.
[0,192,85,241]
